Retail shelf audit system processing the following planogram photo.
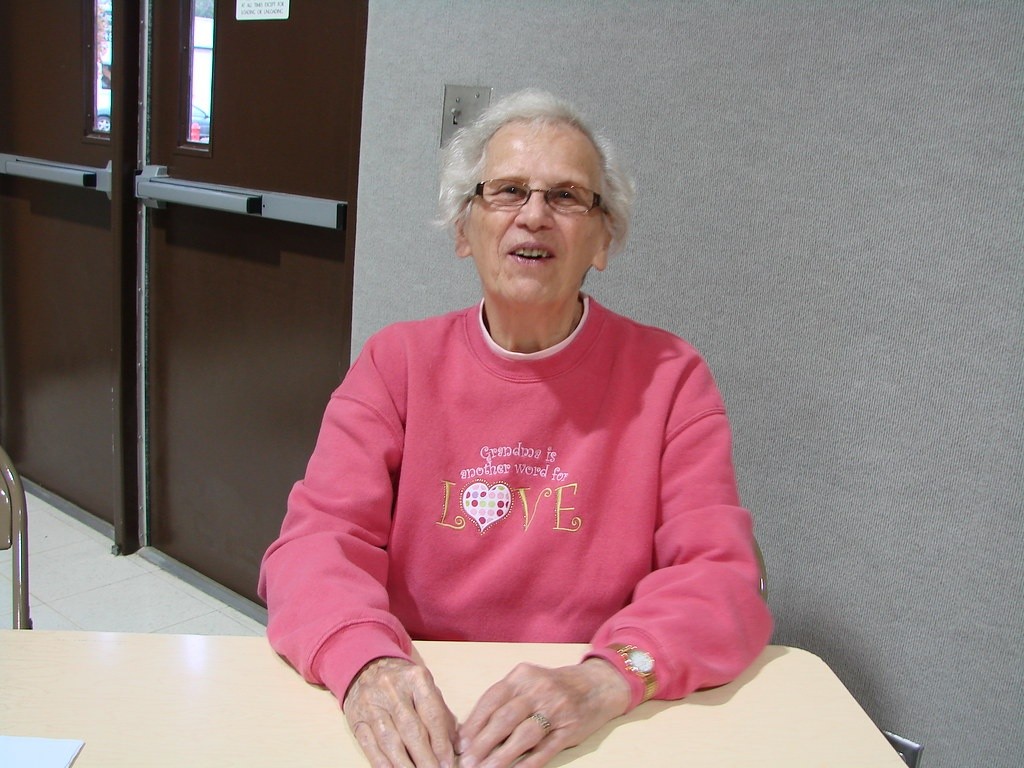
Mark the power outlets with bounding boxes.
[882,731,924,768]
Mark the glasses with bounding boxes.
[461,178,607,209]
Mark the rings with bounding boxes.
[529,714,552,736]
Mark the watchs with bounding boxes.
[604,641,658,704]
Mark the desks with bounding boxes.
[1,619,907,768]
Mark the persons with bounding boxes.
[254,88,774,768]
